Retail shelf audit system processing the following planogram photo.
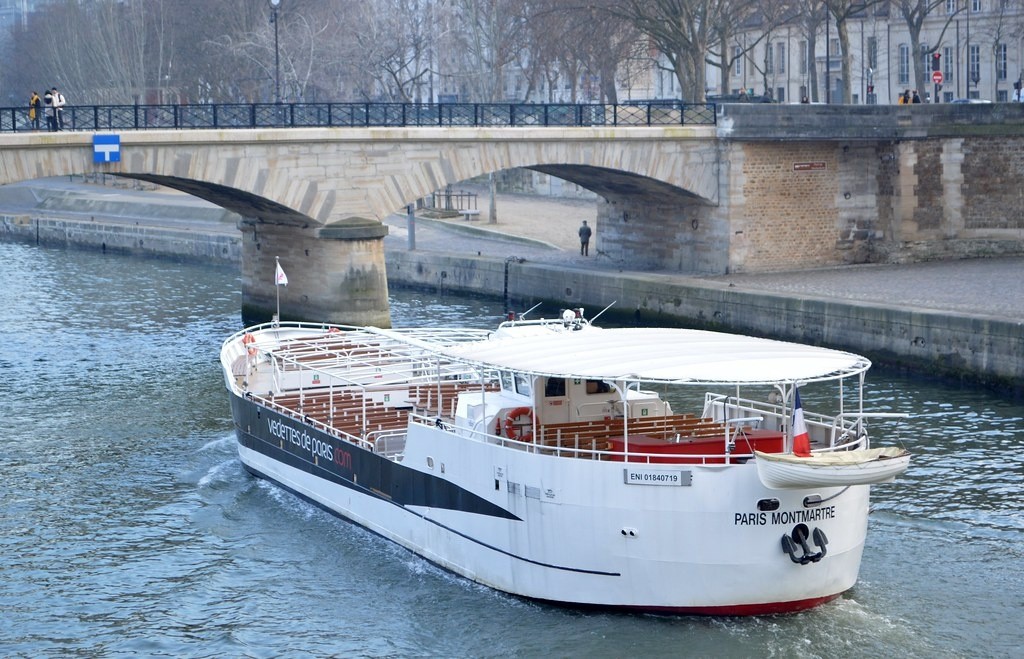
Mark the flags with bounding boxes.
[275,261,288,285]
[792,387,812,457]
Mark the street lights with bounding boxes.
[267,0,283,127]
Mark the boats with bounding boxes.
[219,255,911,618]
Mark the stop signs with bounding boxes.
[932,71,943,84]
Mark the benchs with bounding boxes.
[531,413,754,463]
[249,333,500,455]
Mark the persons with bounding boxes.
[903,89,921,104]
[578,220,592,257]
[28,87,66,133]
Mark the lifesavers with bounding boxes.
[505,406,540,443]
[327,328,342,337]
[243,333,258,356]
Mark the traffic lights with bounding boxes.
[868,86,874,92]
[932,53,941,71]
